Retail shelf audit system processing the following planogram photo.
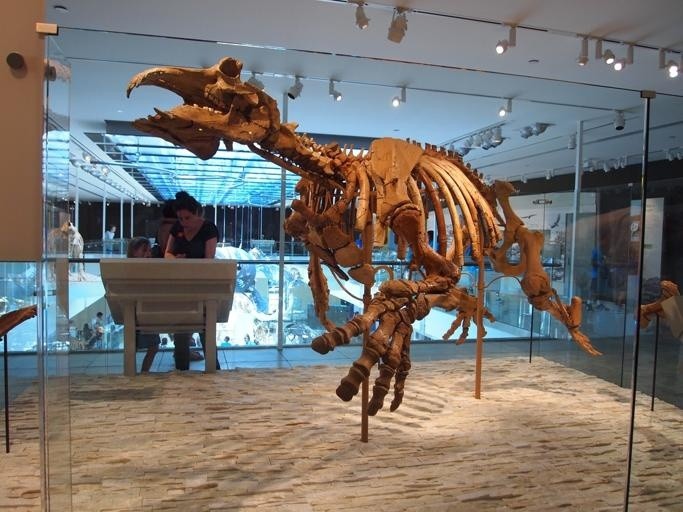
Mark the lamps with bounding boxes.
[520,123,548,139]
[287,82,304,99]
[567,135,576,151]
[614,117,625,131]
[668,70,679,77]
[393,95,400,107]
[446,126,504,157]
[329,90,342,102]
[247,77,265,90]
[498,107,509,121]
[329,0,683,79]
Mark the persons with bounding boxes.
[126,235,163,371]
[587,236,611,311]
[72,307,126,351]
[166,190,206,362]
[105,225,117,257]
[286,267,309,320]
[163,195,222,371]
[156,334,267,346]
[234,247,274,315]
